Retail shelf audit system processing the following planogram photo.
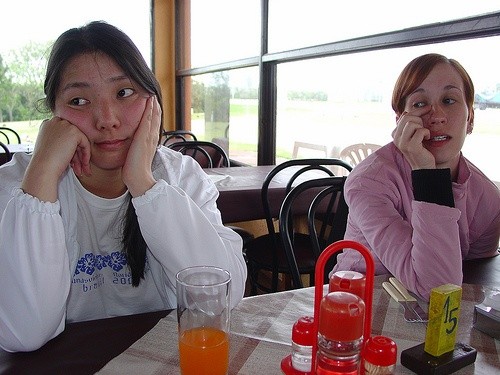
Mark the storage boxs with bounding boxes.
[423,282,462,357]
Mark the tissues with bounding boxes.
[473,290,500,340]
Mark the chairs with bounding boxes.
[161,130,381,296]
[0,127,21,144]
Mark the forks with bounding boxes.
[382,282,425,323]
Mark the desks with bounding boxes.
[0,255,500,375]
[202,164,341,290]
[160,139,226,168]
[0,144,34,163]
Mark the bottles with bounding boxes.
[363,337,398,375]
[316,291,365,375]
[329,271,367,299]
[291,316,314,373]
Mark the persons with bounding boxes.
[0,20,248,352]
[327,53,500,304]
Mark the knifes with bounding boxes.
[389,277,429,328]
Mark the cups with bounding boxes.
[176,267,231,375]
